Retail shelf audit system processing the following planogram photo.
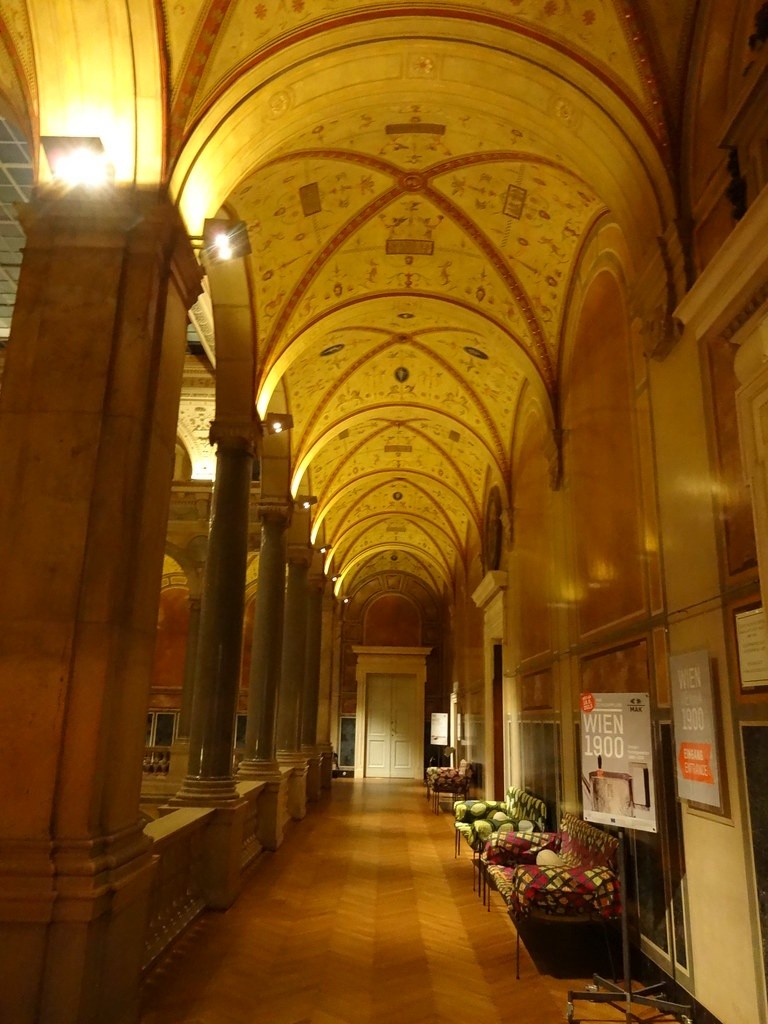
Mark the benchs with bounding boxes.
[452,787,547,857]
[426,760,474,814]
[482,813,621,980]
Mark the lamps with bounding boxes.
[312,544,331,553]
[294,495,317,510]
[326,573,341,581]
[338,595,351,605]
[190,218,251,265]
[261,412,294,435]
[39,135,114,184]
